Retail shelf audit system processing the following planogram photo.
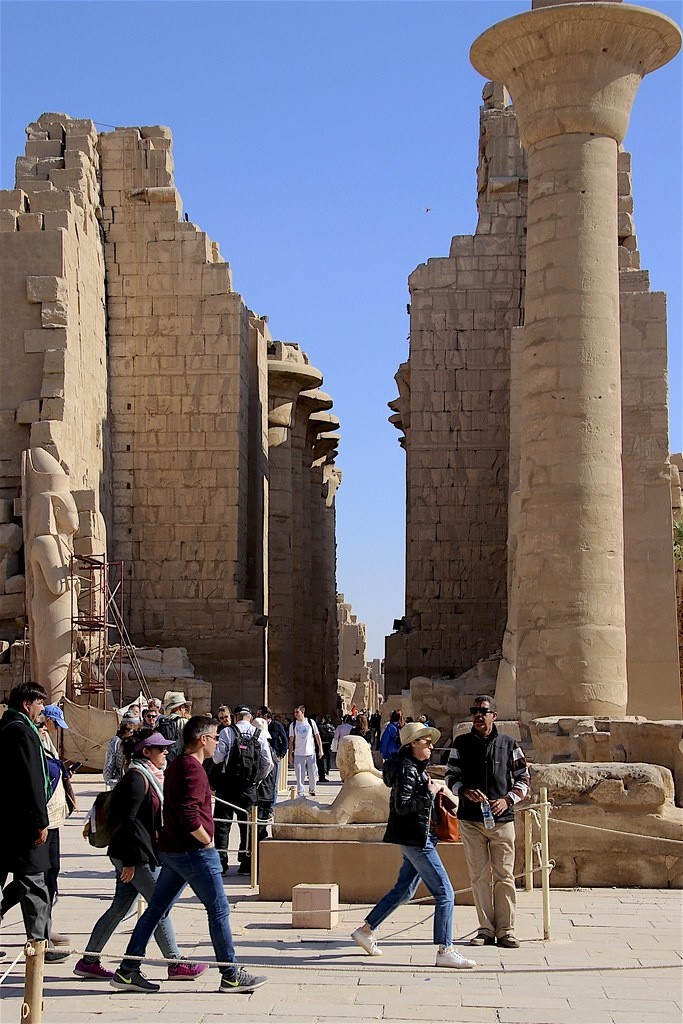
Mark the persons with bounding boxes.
[350,720,477,969]
[73,728,209,980]
[211,705,274,877]
[0,680,73,964]
[444,694,531,948]
[28,449,81,704]
[314,709,434,783]
[289,704,324,797]
[282,718,291,744]
[33,705,75,946]
[102,690,288,848]
[110,713,269,993]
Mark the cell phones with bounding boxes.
[67,761,82,775]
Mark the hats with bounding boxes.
[135,733,176,751]
[122,713,139,724]
[233,705,252,715]
[398,722,441,751]
[164,693,192,709]
[41,705,68,728]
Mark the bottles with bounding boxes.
[480,800,495,829]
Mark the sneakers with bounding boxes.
[436,948,476,968]
[351,927,383,955]
[168,956,209,979]
[73,959,115,979]
[219,965,268,992]
[44,951,72,963]
[0,951,7,960]
[110,968,160,993]
[49,932,71,946]
[470,933,495,946]
[497,933,520,948]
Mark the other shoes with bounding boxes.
[320,777,328,782]
[237,868,251,875]
[221,871,226,876]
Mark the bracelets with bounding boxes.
[203,837,213,845]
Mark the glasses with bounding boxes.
[197,735,220,741]
[417,736,431,746]
[49,718,60,729]
[470,707,495,715]
[146,715,157,718]
[149,746,167,753]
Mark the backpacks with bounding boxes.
[225,724,261,785]
[430,791,462,842]
[82,769,149,848]
